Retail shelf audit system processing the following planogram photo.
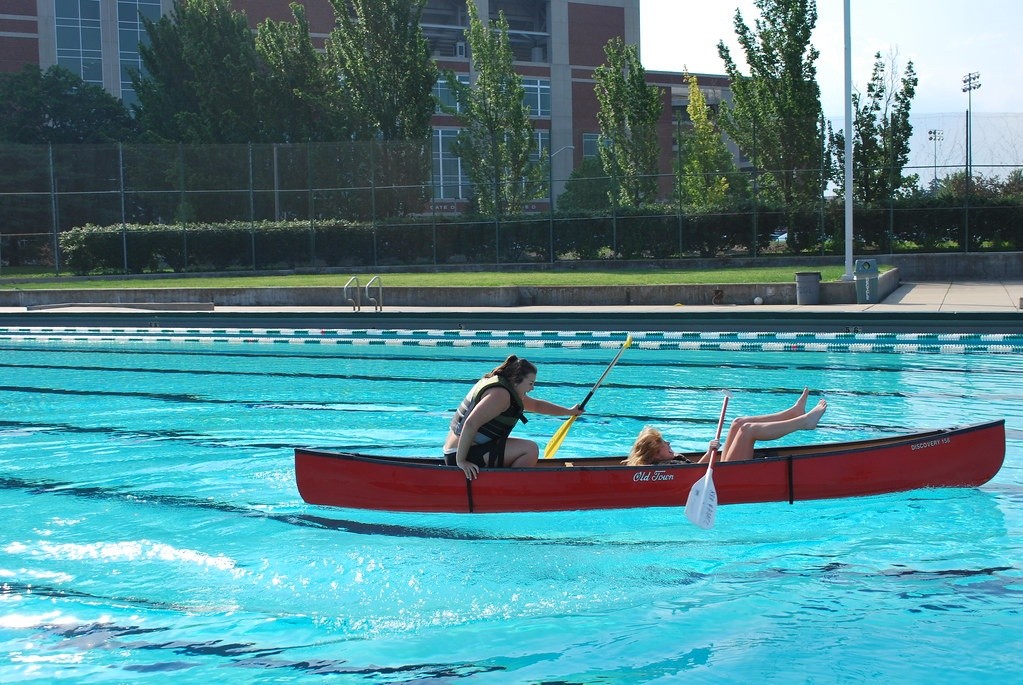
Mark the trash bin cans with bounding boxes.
[795,271,823,306]
[854,259,880,304]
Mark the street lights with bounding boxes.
[927,129,944,180]
[548,144,575,269]
[961,71,981,180]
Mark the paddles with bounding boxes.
[684,390,732,530]
[544,336,632,458]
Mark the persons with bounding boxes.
[442,355,586,481]
[620,385,827,468]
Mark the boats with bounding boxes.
[293,416,1009,516]
